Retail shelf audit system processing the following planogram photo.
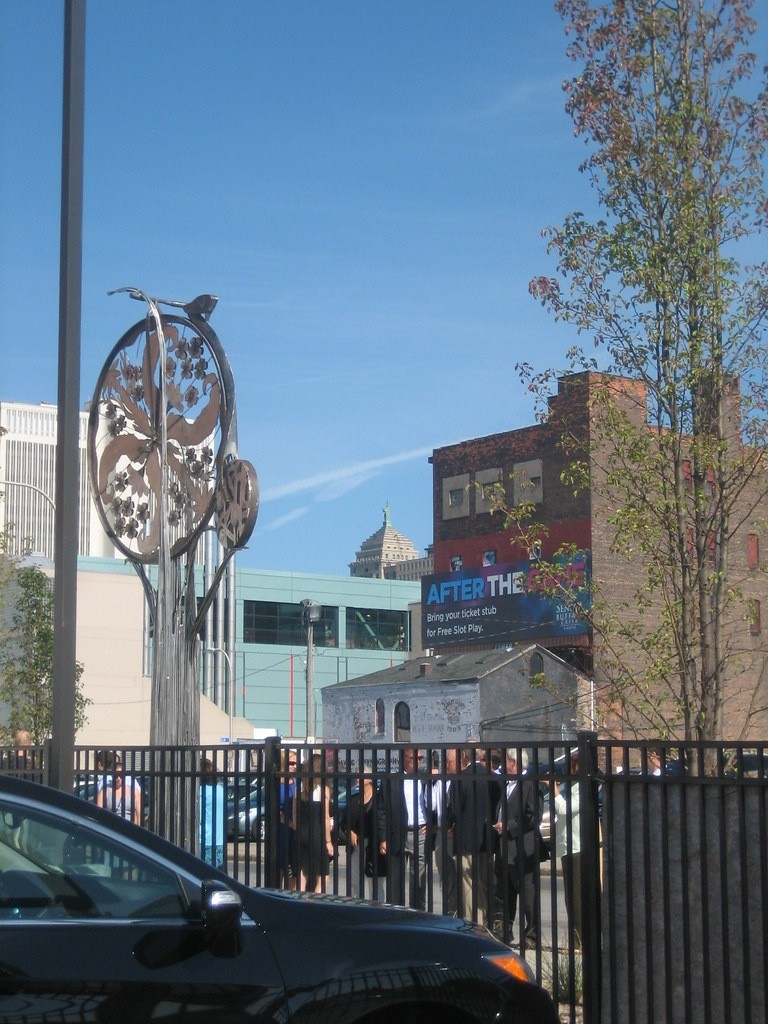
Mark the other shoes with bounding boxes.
[515,938,535,951]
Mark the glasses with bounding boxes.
[289,762,296,765]
[410,756,424,761]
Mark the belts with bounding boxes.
[408,824,427,832]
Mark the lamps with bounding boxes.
[305,604,321,623]
[299,599,313,620]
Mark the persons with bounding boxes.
[0,729,677,956]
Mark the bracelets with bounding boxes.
[326,841,332,843]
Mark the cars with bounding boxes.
[69,776,152,841]
[223,771,269,845]
[0,775,559,1024]
[328,772,385,848]
